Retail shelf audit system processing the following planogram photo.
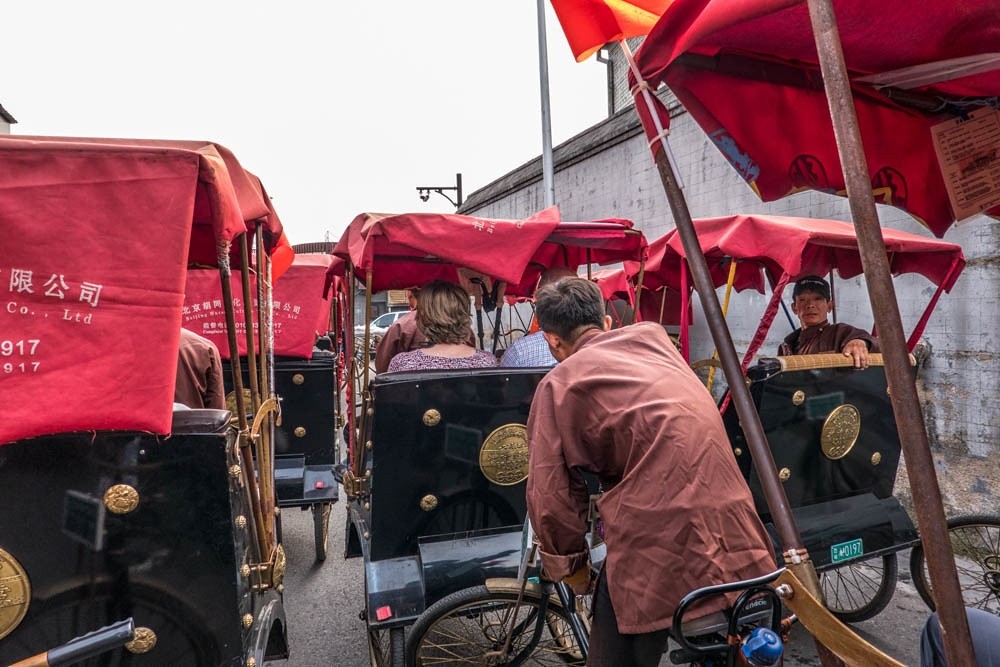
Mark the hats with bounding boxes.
[792,275,830,302]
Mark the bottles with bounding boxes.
[735,626,784,667]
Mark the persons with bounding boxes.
[527,280,781,667]
[374,284,476,372]
[386,280,499,374]
[777,272,883,373]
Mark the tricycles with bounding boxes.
[1,120,1000,666]
